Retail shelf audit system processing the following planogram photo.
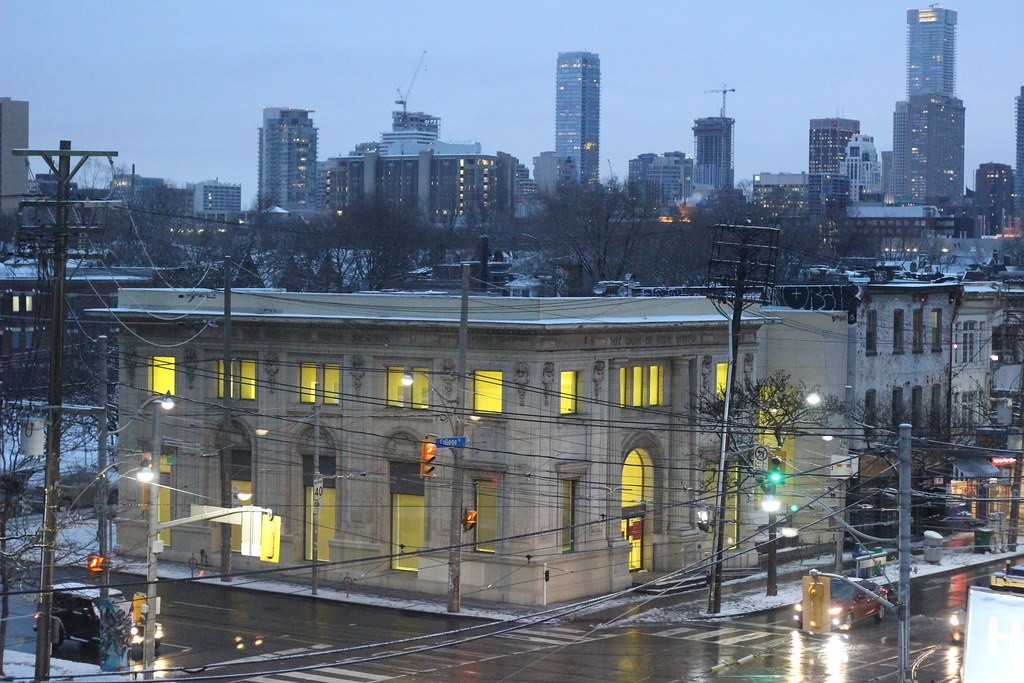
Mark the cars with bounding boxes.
[794,577,888,631]
[32,580,164,651]
[13,454,119,513]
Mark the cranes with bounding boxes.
[393,50,427,112]
[705,83,736,118]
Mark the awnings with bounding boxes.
[953,459,1000,481]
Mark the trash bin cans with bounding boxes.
[974,527,994,554]
[852,543,887,579]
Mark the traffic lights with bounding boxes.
[769,471,782,483]
[88,555,103,571]
[420,439,437,478]
[463,510,478,532]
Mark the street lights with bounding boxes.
[399,366,465,611]
[696,460,728,614]
[110,400,275,681]
[760,495,798,597]
[96,334,177,669]
[807,392,912,683]
[254,381,321,594]
